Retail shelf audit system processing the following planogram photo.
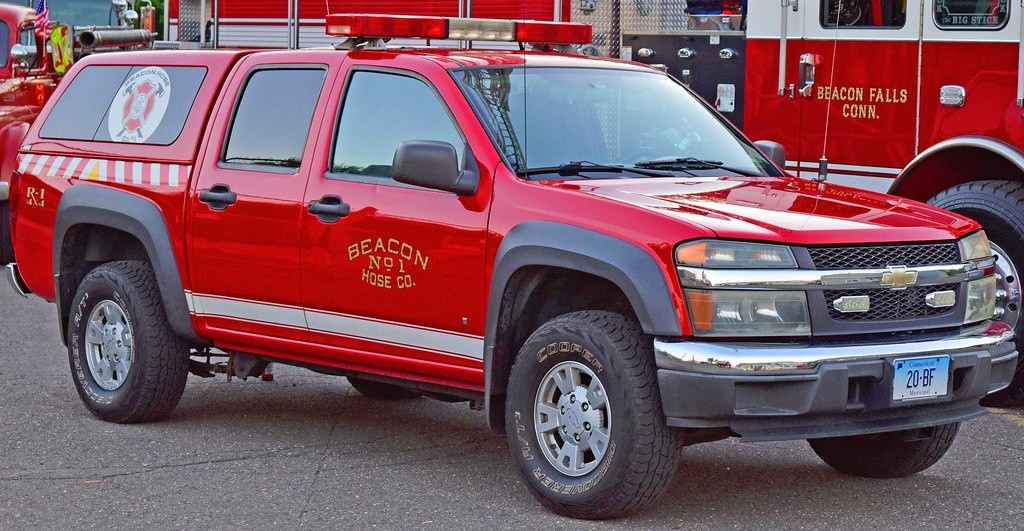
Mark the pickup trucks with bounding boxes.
[8,13,1018,517]
[0,3,69,200]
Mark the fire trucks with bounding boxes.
[164,0,1024,406]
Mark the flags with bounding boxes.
[32,0,53,38]
